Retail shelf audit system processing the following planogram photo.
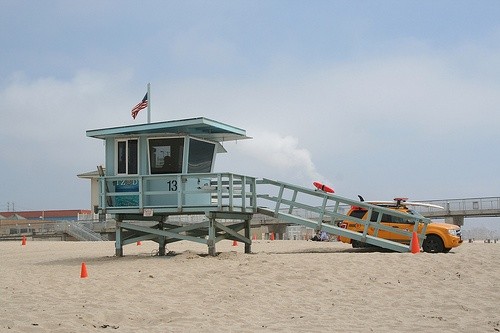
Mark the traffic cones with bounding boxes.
[271,231,274,240]
[232,240,238,246]
[412,232,419,253]
[137,241,142,245]
[81,263,88,278]
[22,235,26,245]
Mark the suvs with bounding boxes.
[338,204,464,253]
